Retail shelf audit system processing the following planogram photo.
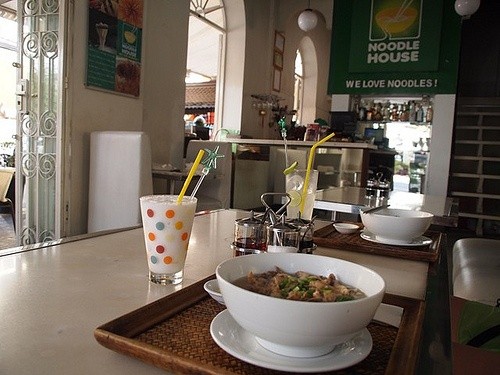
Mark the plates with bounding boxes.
[210,308,372,372]
[360,232,432,246]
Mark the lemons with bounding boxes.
[283,161,299,175]
[286,190,302,206]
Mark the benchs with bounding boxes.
[448,239,500,375]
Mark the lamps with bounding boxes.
[455,0,480,17]
[297,0,318,32]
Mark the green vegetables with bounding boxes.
[276,276,353,302]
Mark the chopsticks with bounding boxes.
[363,205,390,214]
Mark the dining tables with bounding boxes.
[0,207,428,375]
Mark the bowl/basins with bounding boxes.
[216,253,386,359]
[204,279,225,305]
[333,223,359,234]
[359,208,434,244]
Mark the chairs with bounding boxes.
[0,168,16,234]
[88,130,153,233]
[176,140,232,212]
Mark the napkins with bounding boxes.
[372,303,403,328]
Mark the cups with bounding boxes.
[139,194,198,285]
[286,169,318,219]
[307,123,319,141]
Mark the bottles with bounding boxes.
[365,180,377,198]
[404,101,424,122]
[359,100,406,121]
[422,95,427,122]
[234,218,267,257]
[287,219,314,254]
[426,106,433,123]
[377,181,389,199]
[266,224,301,253]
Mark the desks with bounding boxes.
[220,138,378,223]
[314,186,460,233]
[152,170,216,195]
